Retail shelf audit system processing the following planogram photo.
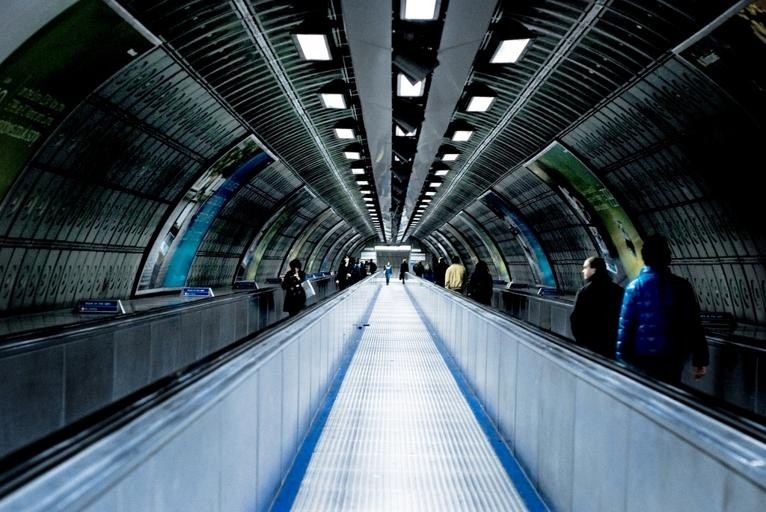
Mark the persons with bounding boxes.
[618,234,709,390]
[614,220,639,259]
[465,260,494,304]
[443,257,466,294]
[567,255,629,365]
[281,258,308,315]
[335,252,450,292]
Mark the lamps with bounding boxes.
[477,19,533,67]
[456,82,497,114]
[318,79,353,111]
[355,179,384,240]
[350,163,366,177]
[437,143,462,165]
[444,118,475,143]
[291,20,338,65]
[389,0,451,246]
[341,143,363,161]
[333,117,359,141]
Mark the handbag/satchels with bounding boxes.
[301,280,316,297]
